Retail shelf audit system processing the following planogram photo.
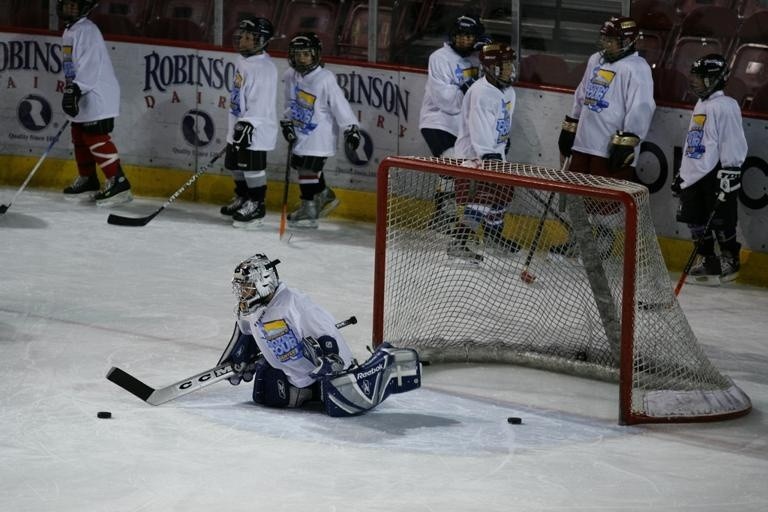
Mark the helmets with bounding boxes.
[450,17,484,57]
[599,16,639,62]
[479,42,516,86]
[687,54,730,102]
[56,0,91,24]
[231,255,278,315]
[288,32,322,72]
[233,17,273,57]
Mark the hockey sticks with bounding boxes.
[108,146,227,226]
[638,192,724,312]
[107,315,357,406]
[520,156,568,284]
[0,119,69,214]
[279,141,293,237]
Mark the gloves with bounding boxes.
[62,82,81,117]
[557,115,579,159]
[712,170,742,203]
[482,153,503,187]
[345,125,361,150]
[280,119,298,145]
[460,74,478,95]
[672,173,685,195]
[233,121,253,147]
[611,130,640,173]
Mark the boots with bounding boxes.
[688,241,741,275]
[287,172,336,221]
[63,173,100,193]
[430,191,615,264]
[94,163,130,200]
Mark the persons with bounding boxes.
[218,16,279,222]
[279,31,360,222]
[444,40,521,264]
[544,14,655,261]
[667,53,746,277]
[56,0,134,202]
[217,254,421,417]
[418,13,485,237]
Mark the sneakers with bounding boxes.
[220,196,266,221]
